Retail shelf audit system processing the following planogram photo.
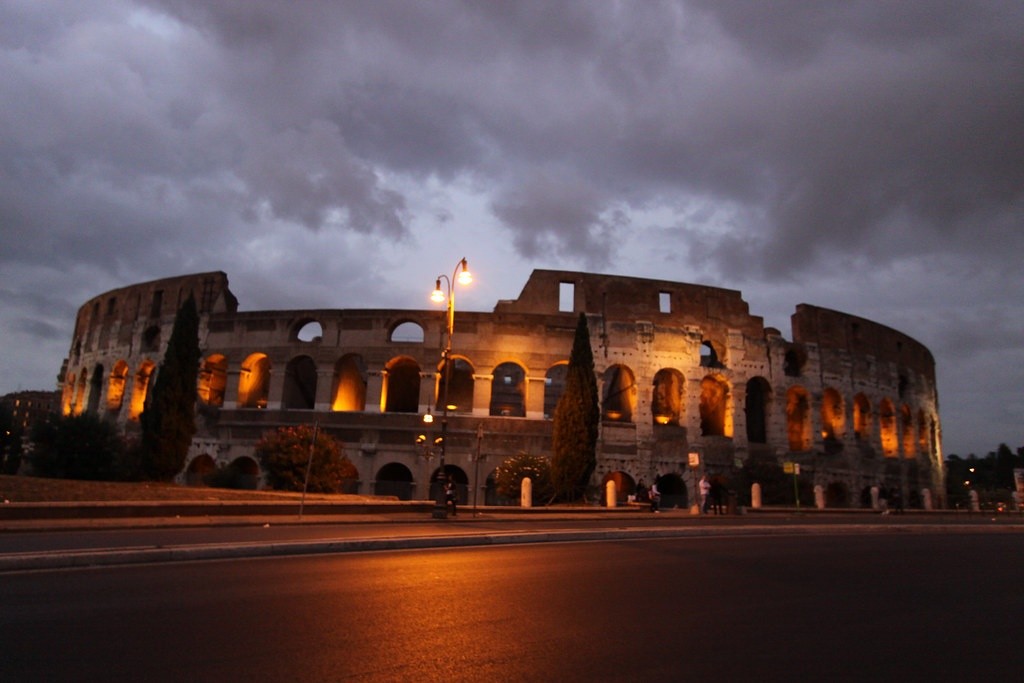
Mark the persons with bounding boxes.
[444,475,457,517]
[636,469,906,516]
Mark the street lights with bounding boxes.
[430,257,474,520]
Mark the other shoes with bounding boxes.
[655,510,659,513]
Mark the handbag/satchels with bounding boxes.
[653,496,660,502]
